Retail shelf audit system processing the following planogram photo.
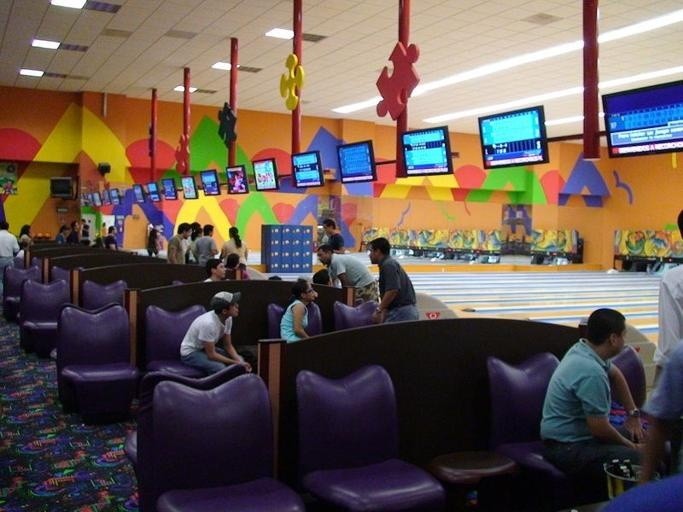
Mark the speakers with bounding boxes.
[98,162,111,173]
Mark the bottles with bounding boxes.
[612,459,638,480]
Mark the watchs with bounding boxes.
[628,407,640,417]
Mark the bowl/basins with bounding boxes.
[604,464,645,500]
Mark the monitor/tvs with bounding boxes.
[180,176,199,200]
[50,176,72,198]
[200,169,220,196]
[252,158,279,191]
[601,79,683,158]
[401,125,454,177]
[336,139,378,184]
[87,177,177,207]
[291,151,325,188]
[226,164,249,195]
[478,105,549,170]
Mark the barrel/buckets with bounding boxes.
[604,459,660,499]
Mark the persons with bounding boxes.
[18,224,35,249]
[179,291,252,375]
[0,221,21,314]
[279,219,419,344]
[638,339,683,482]
[55,219,250,282]
[540,308,672,477]
[652,209,682,382]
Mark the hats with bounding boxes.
[209,291,242,310]
